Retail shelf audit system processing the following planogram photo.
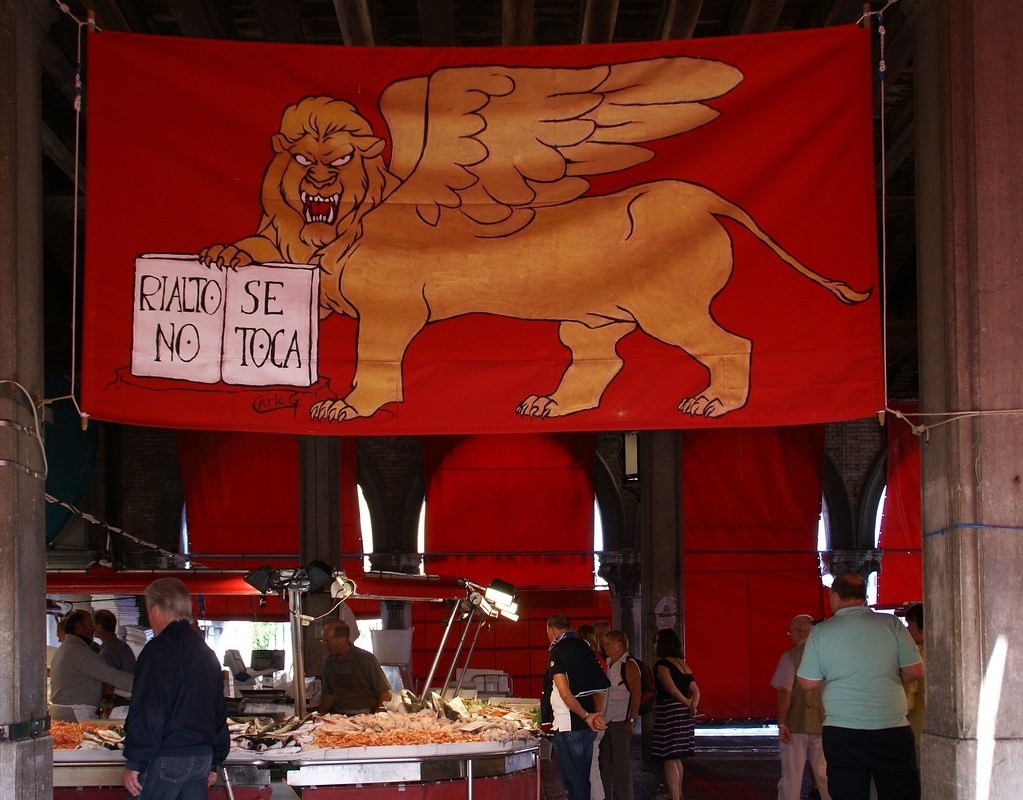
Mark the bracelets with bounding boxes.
[778,723,785,728]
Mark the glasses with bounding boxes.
[787,629,800,636]
[320,639,341,646]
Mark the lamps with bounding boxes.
[485,579,520,623]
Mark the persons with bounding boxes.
[540,612,700,800]
[797,574,925,800]
[775,614,832,800]
[899,599,924,751]
[320,621,393,719]
[122,577,230,800]
[47,608,137,725]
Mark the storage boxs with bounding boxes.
[315,680,321,693]
[254,669,278,688]
[233,675,263,698]
[221,666,234,697]
[225,650,246,675]
[289,681,312,700]
[489,697,539,712]
[370,630,412,665]
[305,677,317,695]
[251,650,285,670]
